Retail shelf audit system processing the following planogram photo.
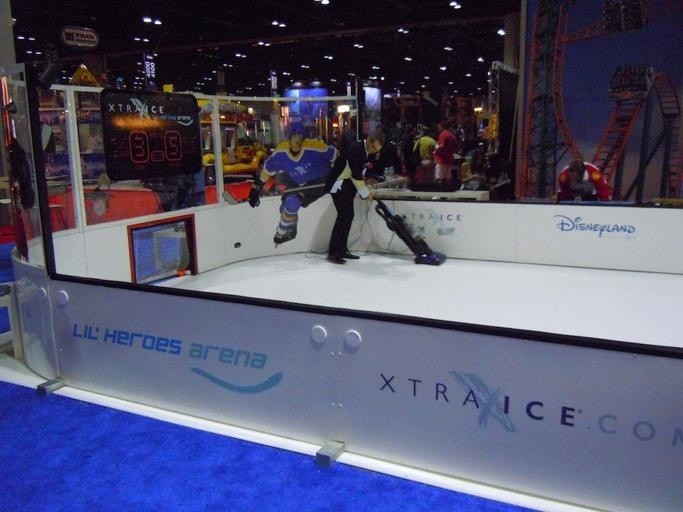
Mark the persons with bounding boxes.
[249,121,515,264]
[557,159,613,203]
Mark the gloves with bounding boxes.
[247,184,262,207]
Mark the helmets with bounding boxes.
[284,121,306,141]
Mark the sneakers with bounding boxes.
[274,233,296,243]
[328,249,360,262]
